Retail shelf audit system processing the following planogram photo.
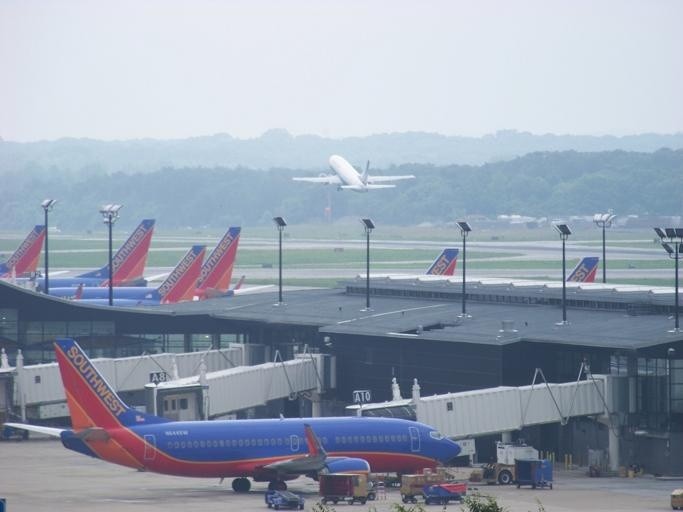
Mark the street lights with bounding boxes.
[97,204,119,309]
[591,212,618,284]
[271,216,289,309]
[553,220,576,324]
[40,197,60,295]
[652,225,683,330]
[457,218,474,318]
[358,216,376,311]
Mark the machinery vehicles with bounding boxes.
[480,440,540,485]
[260,472,466,508]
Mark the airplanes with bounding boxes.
[290,156,416,194]
[0,217,240,302]
[2,339,461,491]
[424,247,457,277]
[566,258,601,286]
